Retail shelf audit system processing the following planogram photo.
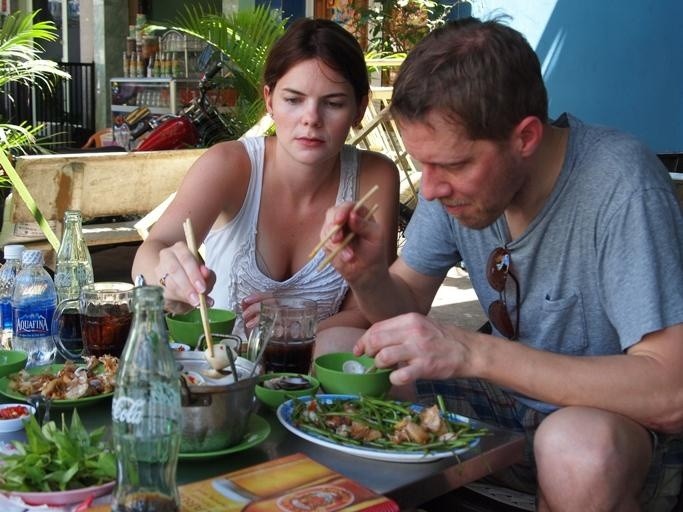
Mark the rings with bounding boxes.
[156,271,171,286]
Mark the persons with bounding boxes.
[123,14,398,332]
[317,17,681,511]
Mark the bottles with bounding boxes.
[110,284,184,510]
[0,244,25,351]
[52,209,95,360]
[112,14,237,110]
[12,249,57,367]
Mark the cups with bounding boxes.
[49,280,134,365]
[259,295,319,377]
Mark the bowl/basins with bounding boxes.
[253,372,321,410]
[0,442,116,509]
[164,309,237,350]
[1,349,28,378]
[313,351,395,401]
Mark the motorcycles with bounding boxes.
[0,44,237,188]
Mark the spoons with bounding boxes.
[341,360,378,375]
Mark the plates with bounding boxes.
[0,403,37,435]
[1,360,116,411]
[274,393,483,464]
[177,406,273,462]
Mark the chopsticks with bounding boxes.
[181,218,216,362]
[308,184,381,273]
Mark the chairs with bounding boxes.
[465,152,683,512]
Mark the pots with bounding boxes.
[170,332,258,453]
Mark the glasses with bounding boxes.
[486,245,521,341]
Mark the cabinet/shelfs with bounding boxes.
[110,33,205,127]
